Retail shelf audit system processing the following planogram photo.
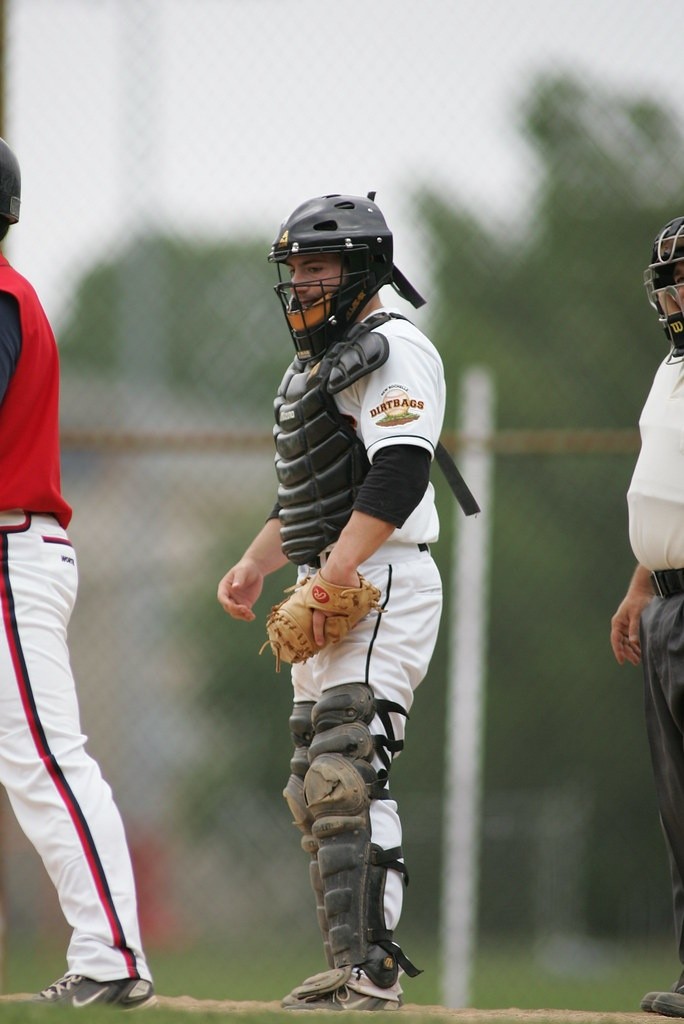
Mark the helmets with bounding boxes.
[268,192,426,364]
[0,137,21,225]
[642,216,684,366]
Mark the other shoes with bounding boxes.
[281,984,406,1012]
[640,990,684,1017]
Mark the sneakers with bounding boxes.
[33,971,158,1013]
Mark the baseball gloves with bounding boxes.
[265,564,383,666]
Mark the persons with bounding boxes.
[211,189,447,1009]
[609,217,684,1016]
[0,137,159,1010]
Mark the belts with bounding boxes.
[650,569,684,598]
[304,542,429,569]
